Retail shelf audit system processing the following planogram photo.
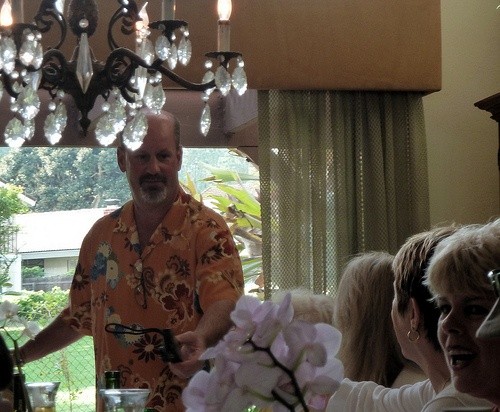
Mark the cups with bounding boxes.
[99,388,151,412]
[24,381,61,412]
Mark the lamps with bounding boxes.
[0,0,248,151]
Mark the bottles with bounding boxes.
[104,370,120,389]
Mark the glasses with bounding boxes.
[488,269,500,296]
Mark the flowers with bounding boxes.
[181,291,345,412]
[0,300,42,372]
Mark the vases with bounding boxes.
[13,374,32,412]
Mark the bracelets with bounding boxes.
[15,348,25,368]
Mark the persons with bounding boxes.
[6,109,244,412]
[272,217,500,412]
